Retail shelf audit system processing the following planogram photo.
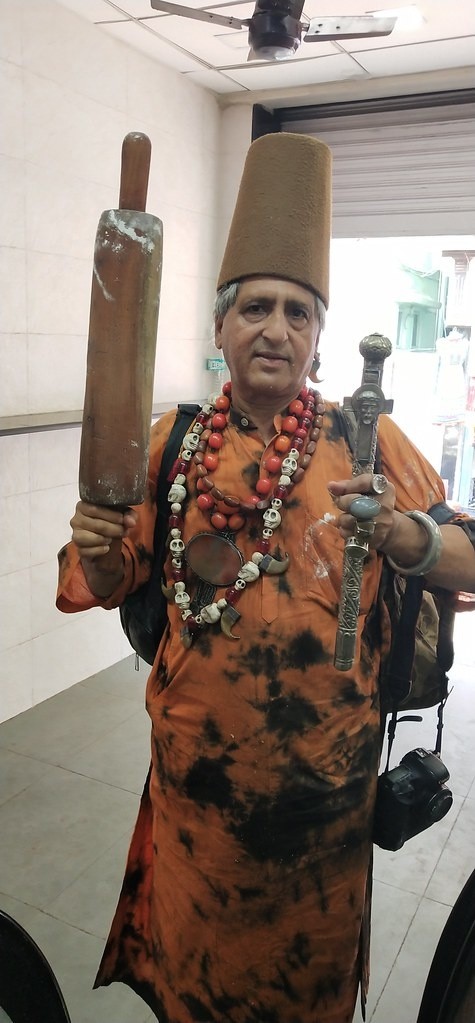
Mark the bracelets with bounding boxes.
[383,509,443,578]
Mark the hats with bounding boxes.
[215,132,334,311]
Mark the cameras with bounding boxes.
[373,746,454,852]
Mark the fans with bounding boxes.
[148,0,399,62]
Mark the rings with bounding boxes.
[342,535,370,559]
[365,473,388,495]
[349,517,376,539]
[346,495,382,518]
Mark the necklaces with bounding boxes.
[196,378,323,528]
[159,382,318,648]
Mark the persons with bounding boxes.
[55,131,475,1021]
